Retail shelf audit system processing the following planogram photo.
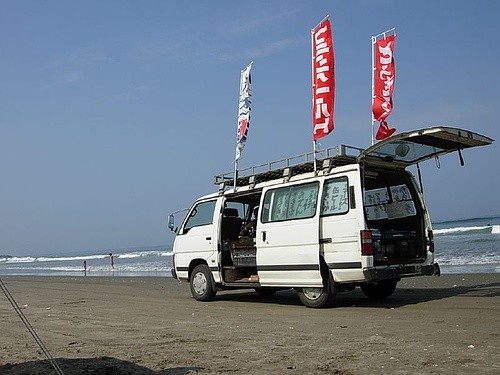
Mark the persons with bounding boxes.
[108,252,115,269]
[82,260,87,276]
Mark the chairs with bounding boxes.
[220,207,243,245]
[238,205,259,237]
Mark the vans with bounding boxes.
[167,125,495,308]
